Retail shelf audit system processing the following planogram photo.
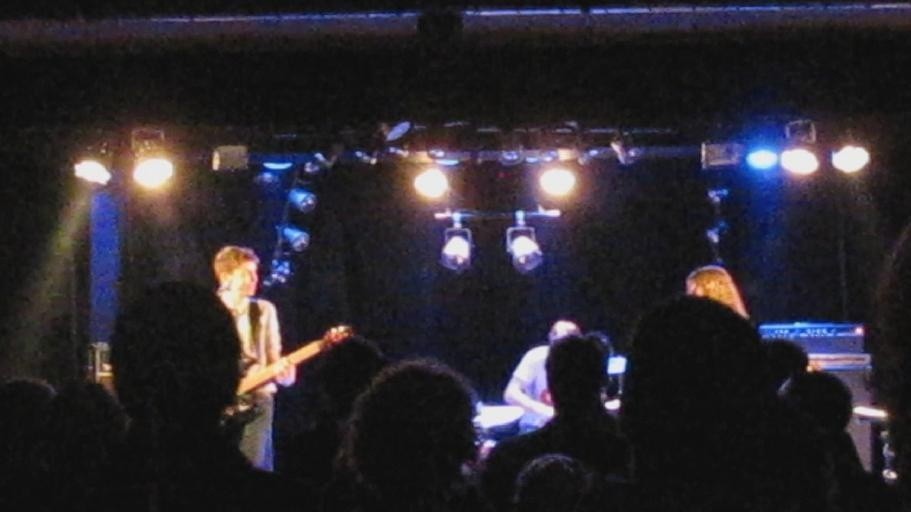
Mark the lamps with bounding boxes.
[433,205,565,278]
[68,122,179,191]
[701,184,737,252]
[274,184,322,258]
[778,111,880,178]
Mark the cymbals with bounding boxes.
[472,406,525,427]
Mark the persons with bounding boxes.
[0,220,911,512]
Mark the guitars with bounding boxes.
[219,324,354,432]
